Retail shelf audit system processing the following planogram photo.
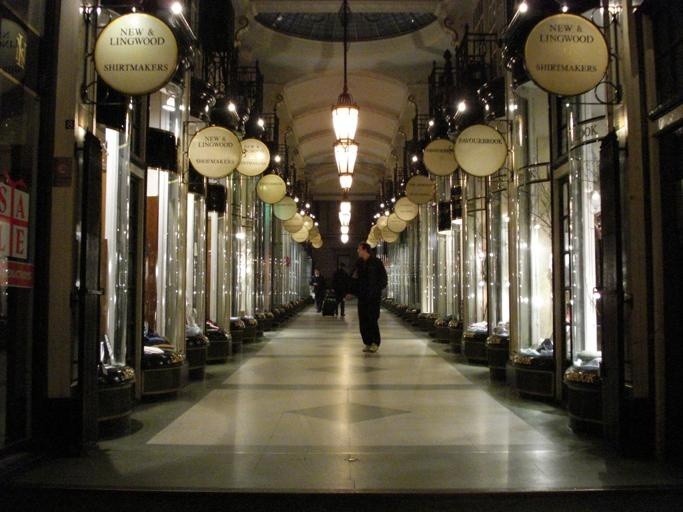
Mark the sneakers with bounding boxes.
[362,342,379,354]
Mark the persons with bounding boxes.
[330,262,349,316]
[309,268,325,313]
[345,243,388,353]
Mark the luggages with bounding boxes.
[321,299,336,317]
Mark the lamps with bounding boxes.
[326,1,360,176]
